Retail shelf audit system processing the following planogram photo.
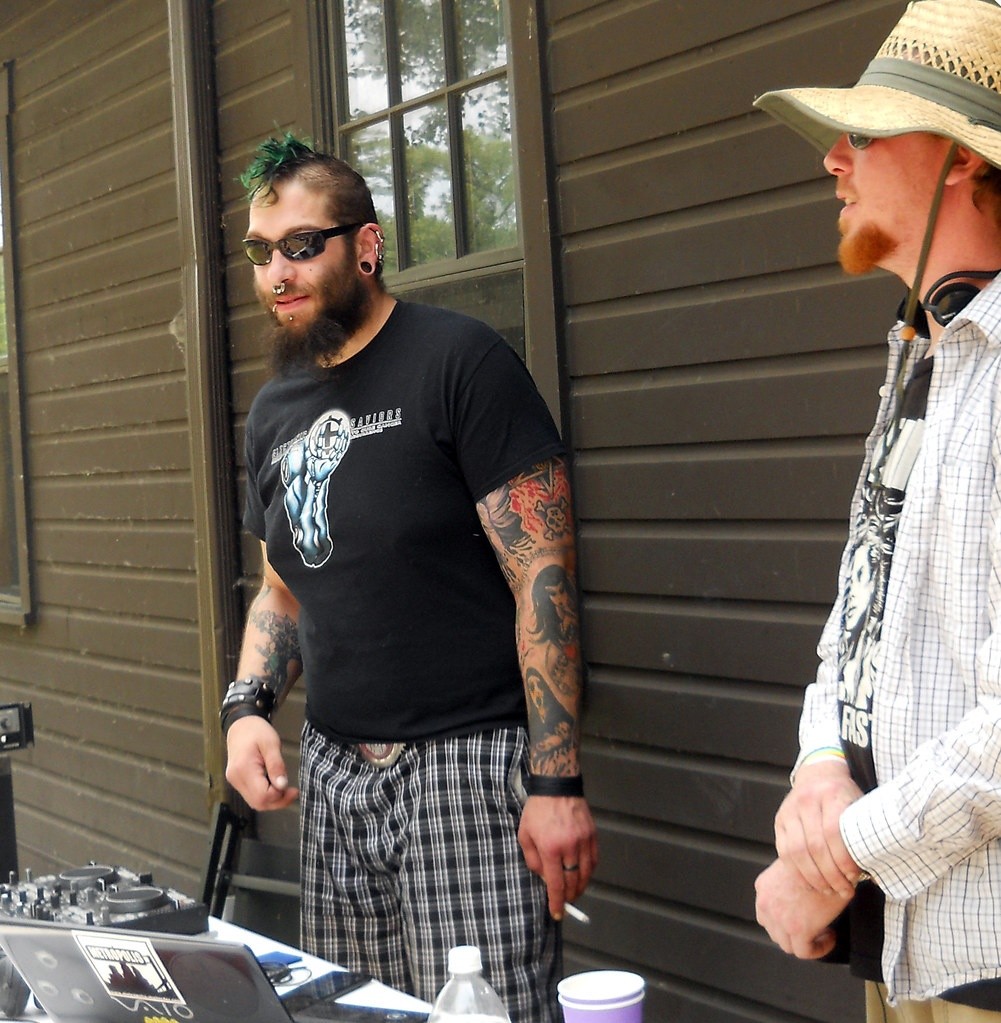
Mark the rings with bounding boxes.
[562,864,580,872]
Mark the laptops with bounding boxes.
[0,919,429,1021]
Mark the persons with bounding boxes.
[222,130,601,1022]
[752,0,1001,1023]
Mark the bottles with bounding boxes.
[426,945,513,1023]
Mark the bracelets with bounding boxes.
[217,678,277,735]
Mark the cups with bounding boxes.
[558,970,645,1023]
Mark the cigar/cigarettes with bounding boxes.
[563,900,590,924]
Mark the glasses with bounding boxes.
[847,133,874,150]
[241,222,358,266]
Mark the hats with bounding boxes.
[751,0,1001,169]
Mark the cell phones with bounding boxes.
[280,971,372,1014]
